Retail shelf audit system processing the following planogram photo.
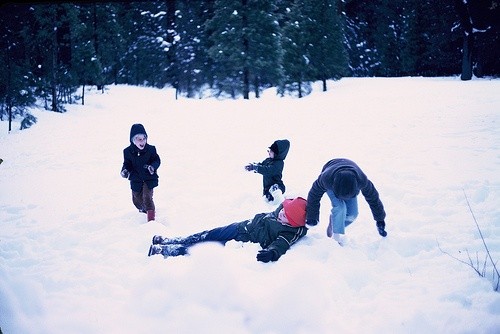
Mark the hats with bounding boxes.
[268,143,278,158]
[334,170,359,200]
[283,196,307,228]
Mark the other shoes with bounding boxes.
[333,233,345,248]
[146,210,155,222]
[327,214,333,238]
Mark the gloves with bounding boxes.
[306,219,320,226]
[376,221,387,237]
[245,163,258,171]
[256,250,273,263]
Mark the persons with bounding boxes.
[120,123,161,222]
[147,197,309,263]
[304,158,388,247]
[244,140,290,202]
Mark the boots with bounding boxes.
[148,243,186,257]
[153,229,208,246]
[269,189,285,206]
